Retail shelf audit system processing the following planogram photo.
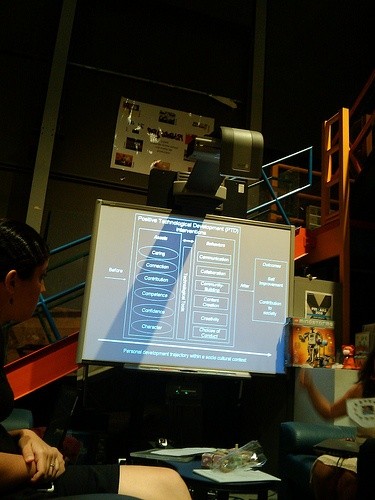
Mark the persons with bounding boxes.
[299,326,375,500]
[296,325,329,364]
[0,218,192,500]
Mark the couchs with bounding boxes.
[277,420,359,500]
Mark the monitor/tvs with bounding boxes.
[76,199,295,379]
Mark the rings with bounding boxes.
[50,464,55,466]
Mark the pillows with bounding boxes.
[314,437,367,458]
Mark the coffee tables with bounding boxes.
[130,447,273,500]
